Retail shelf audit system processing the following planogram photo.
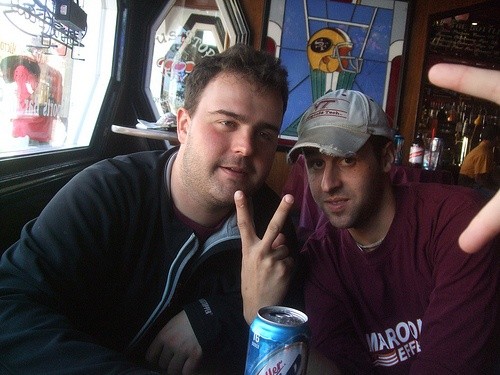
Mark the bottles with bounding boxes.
[409,132,425,167]
[415,95,500,141]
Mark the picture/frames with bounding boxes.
[261,0,416,152]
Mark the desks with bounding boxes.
[111,123,180,150]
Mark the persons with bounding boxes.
[1,43,294,375]
[233,89,500,375]
[457,125,500,190]
[14,38,62,147]
[428,62,500,254]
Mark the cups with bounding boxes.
[423,135,442,171]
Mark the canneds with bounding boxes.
[244,305,309,375]
[393,134,405,165]
[427,137,443,169]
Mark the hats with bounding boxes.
[287,89,392,164]
[26,37,50,47]
